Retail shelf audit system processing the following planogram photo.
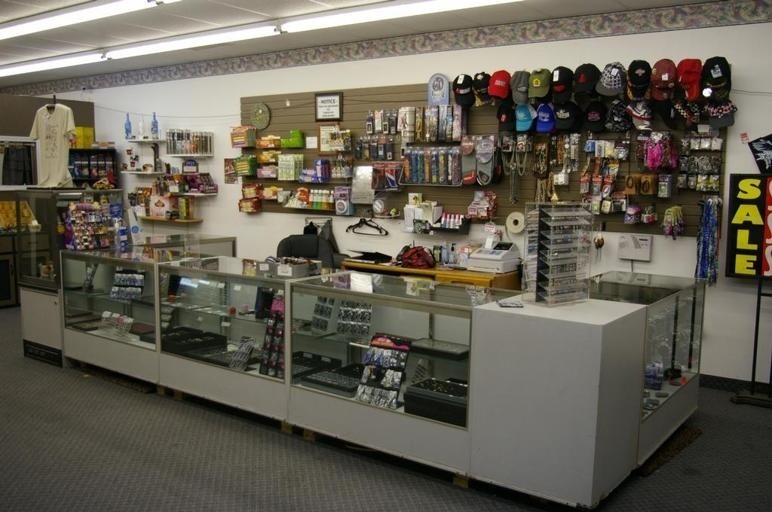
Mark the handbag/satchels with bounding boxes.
[402,247,436,269]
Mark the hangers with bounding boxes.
[345,213,382,233]
[352,209,389,236]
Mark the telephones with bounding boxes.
[396,243,411,261]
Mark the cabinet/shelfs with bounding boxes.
[14,185,126,369]
[289,269,536,498]
[0,136,42,191]
[371,136,464,193]
[241,140,353,215]
[0,234,49,310]
[575,269,709,470]
[68,146,117,189]
[118,136,217,224]
[60,241,159,397]
[156,255,353,438]
[125,232,237,258]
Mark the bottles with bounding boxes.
[136,112,145,138]
[166,129,213,154]
[309,189,335,209]
[123,113,132,139]
[150,112,159,139]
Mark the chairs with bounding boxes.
[276,234,334,270]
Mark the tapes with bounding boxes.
[505,211,527,241]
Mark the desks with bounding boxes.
[342,256,524,290]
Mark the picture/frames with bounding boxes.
[313,91,343,122]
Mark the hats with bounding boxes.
[626,101,652,131]
[550,66,571,103]
[675,102,700,123]
[515,106,536,131]
[572,63,601,93]
[488,70,510,98]
[701,100,736,129]
[554,101,581,129]
[656,103,675,128]
[595,62,626,97]
[536,102,554,133]
[583,101,609,132]
[528,68,549,97]
[702,56,730,104]
[509,71,527,105]
[677,59,701,102]
[627,60,649,100]
[605,100,632,133]
[497,104,513,131]
[453,75,476,107]
[472,72,495,107]
[650,59,677,100]
